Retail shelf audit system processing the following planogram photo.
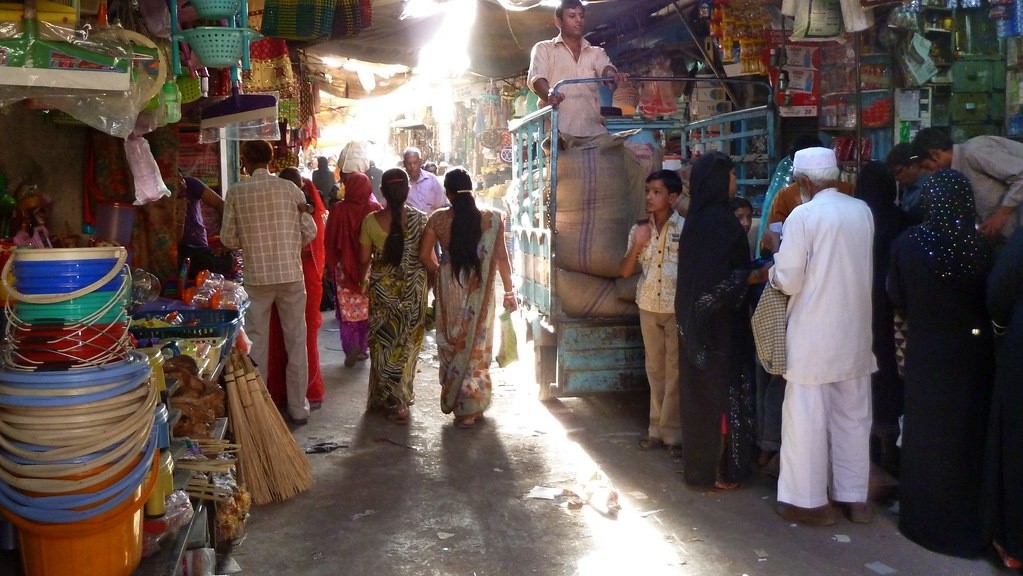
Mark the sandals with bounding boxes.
[474,412,482,420]
[388,408,411,425]
[454,418,474,428]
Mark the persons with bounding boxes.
[262,166,327,415]
[355,169,437,424]
[526,0,628,150]
[620,126,1023,573]
[419,168,519,430]
[219,138,316,422]
[176,173,229,282]
[281,137,453,368]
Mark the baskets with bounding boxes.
[91,28,166,104]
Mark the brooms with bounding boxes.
[224,349,313,506]
[196,14,278,129]
[1,2,133,91]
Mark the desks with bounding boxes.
[0,418,229,576]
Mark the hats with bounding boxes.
[793,147,837,169]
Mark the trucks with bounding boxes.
[500,73,782,404]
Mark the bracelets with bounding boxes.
[504,291,514,296]
[504,295,515,299]
[305,203,310,212]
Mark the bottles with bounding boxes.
[162,80,182,123]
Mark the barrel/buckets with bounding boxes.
[95,202,135,243]
[0,246,160,576]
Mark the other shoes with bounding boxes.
[345,347,359,367]
[311,400,322,408]
[775,502,835,526]
[292,417,310,425]
[852,503,872,522]
[686,482,713,492]
[668,446,681,457]
[637,436,663,450]
[714,481,738,490]
[357,352,369,361]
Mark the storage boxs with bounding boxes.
[688,79,733,151]
[129,309,242,378]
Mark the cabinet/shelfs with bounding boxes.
[815,2,1006,199]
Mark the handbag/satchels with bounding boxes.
[426,306,436,332]
[496,310,519,368]
[894,310,907,375]
